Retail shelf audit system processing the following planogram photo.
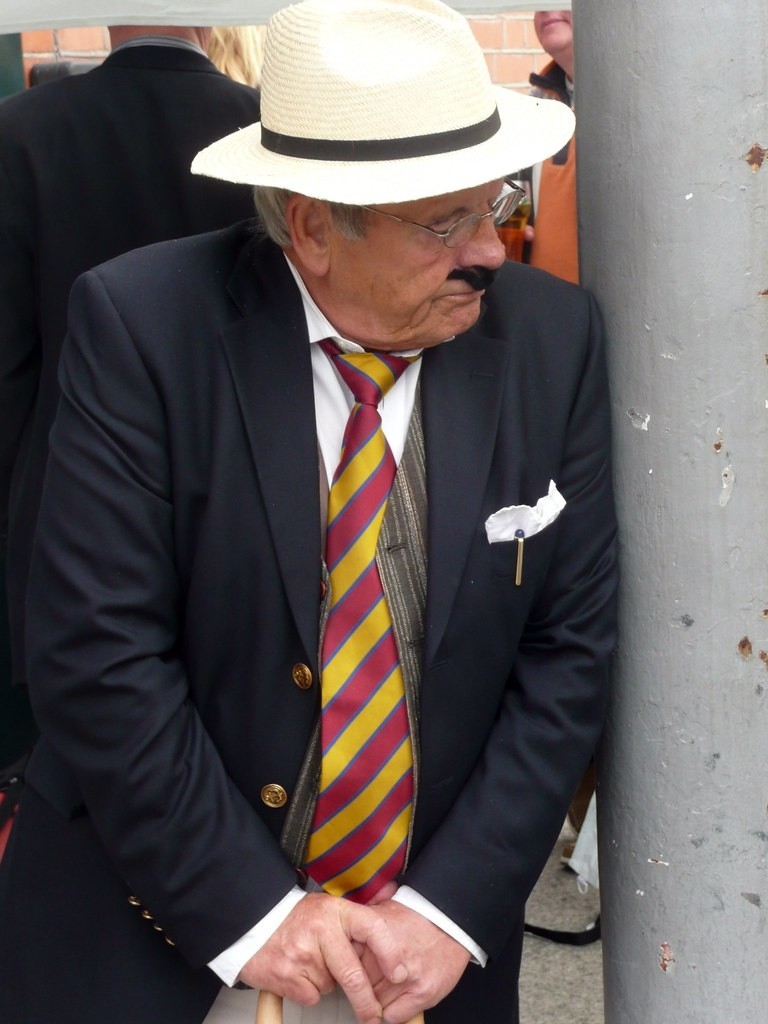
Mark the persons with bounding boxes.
[0,26,260,772]
[524,11,597,864]
[0,0,620,1024]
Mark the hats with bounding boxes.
[189,0,579,208]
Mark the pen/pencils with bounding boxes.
[513,529,525,586]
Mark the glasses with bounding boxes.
[360,179,529,248]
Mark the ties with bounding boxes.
[300,339,427,915]
